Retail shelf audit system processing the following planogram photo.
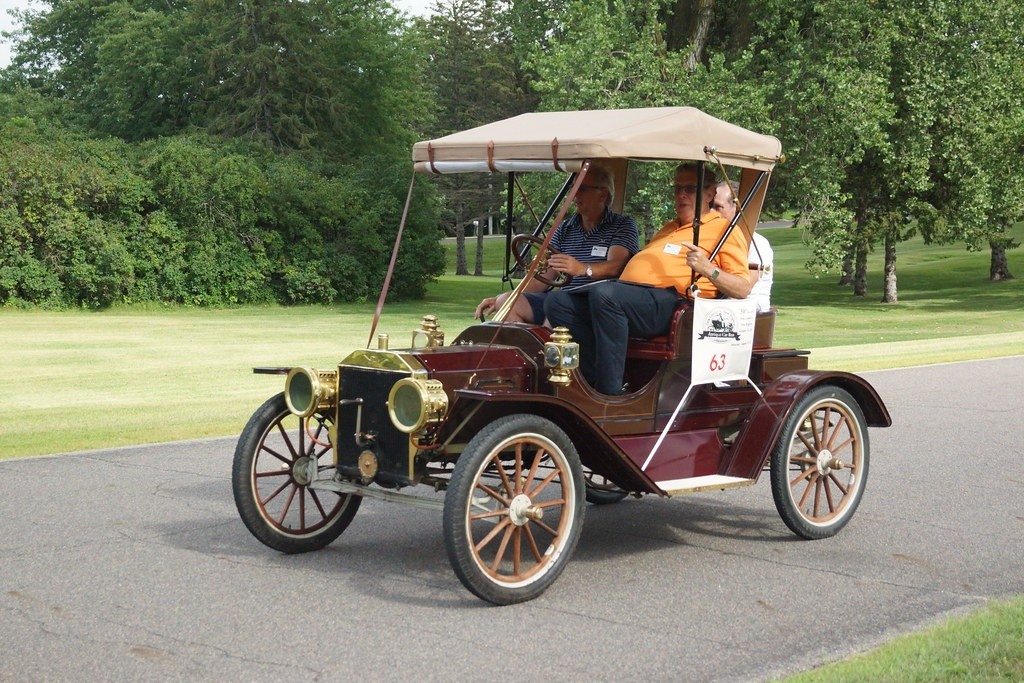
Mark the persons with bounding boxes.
[475,164,638,326]
[544,164,751,396]
[711,180,773,314]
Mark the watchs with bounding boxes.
[707,265,720,281]
[584,262,592,278]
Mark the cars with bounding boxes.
[229,106,892,605]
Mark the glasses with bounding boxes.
[671,186,697,193]
[569,184,602,192]
